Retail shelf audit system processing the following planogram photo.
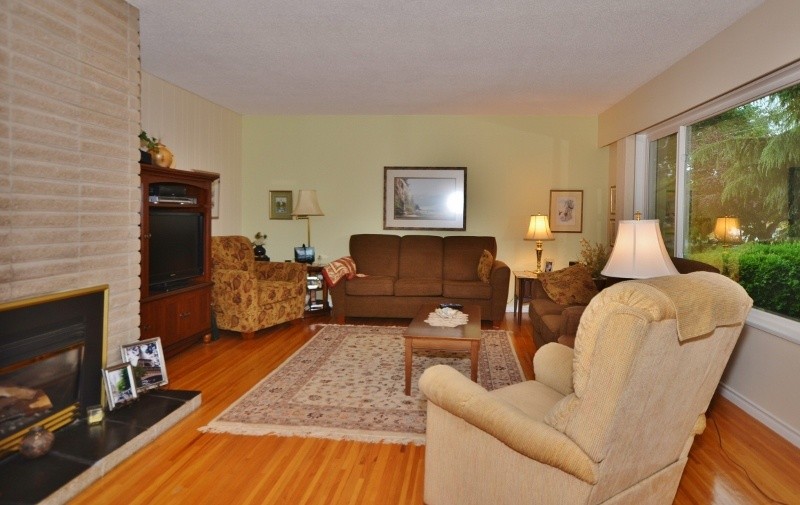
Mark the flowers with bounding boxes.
[251,232,268,246]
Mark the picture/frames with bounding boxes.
[609,185,617,216]
[543,258,554,273]
[548,188,584,234]
[609,218,616,247]
[382,165,468,232]
[100,336,170,413]
[267,189,294,221]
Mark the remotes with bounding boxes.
[441,303,464,309]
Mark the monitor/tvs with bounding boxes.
[147,213,203,293]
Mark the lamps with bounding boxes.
[707,215,746,282]
[522,215,557,277]
[600,210,681,281]
[288,189,325,248]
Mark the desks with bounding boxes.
[512,270,540,327]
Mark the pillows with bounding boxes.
[536,263,600,307]
[475,249,495,283]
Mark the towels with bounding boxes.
[633,270,755,343]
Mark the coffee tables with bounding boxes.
[402,300,483,396]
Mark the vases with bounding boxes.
[253,245,267,257]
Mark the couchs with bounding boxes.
[416,270,755,505]
[211,235,309,342]
[329,234,511,330]
[528,277,588,351]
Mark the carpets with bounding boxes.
[196,322,529,450]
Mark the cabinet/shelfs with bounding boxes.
[304,264,331,319]
[141,165,221,360]
